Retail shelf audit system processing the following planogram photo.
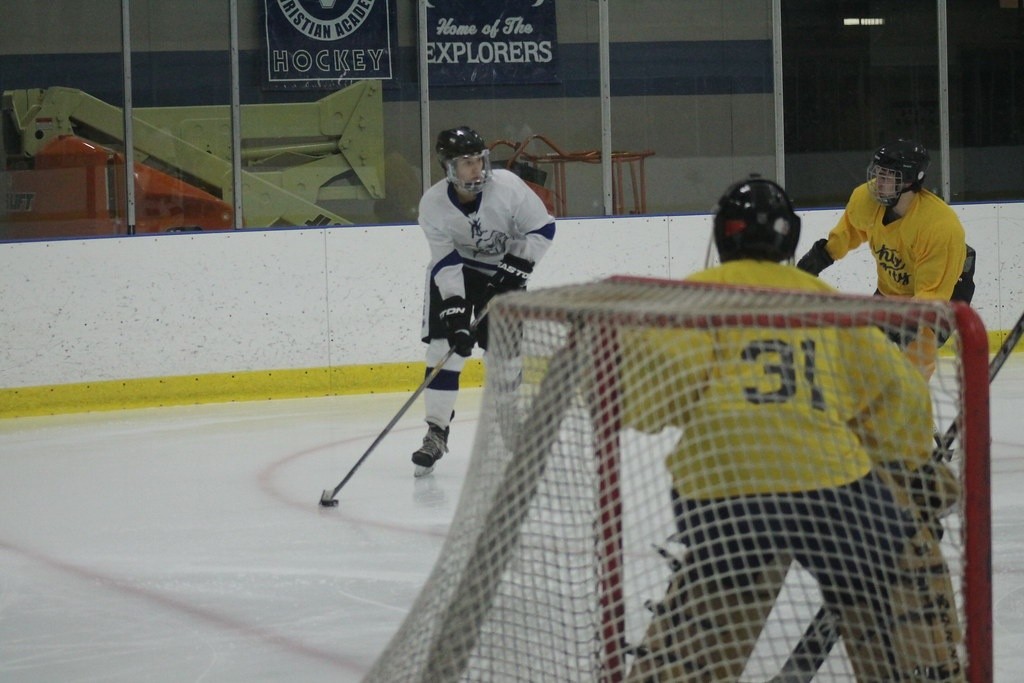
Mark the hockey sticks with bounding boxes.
[763,311,1024,683]
[319,293,499,508]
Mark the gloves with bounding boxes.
[439,295,479,357]
[797,238,835,276]
[482,253,535,302]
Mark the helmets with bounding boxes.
[437,126,493,193]
[714,180,802,263]
[866,139,931,209]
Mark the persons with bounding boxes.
[412,126,556,477]
[578,174,966,683]
[796,140,976,382]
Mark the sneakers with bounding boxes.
[412,410,455,477]
